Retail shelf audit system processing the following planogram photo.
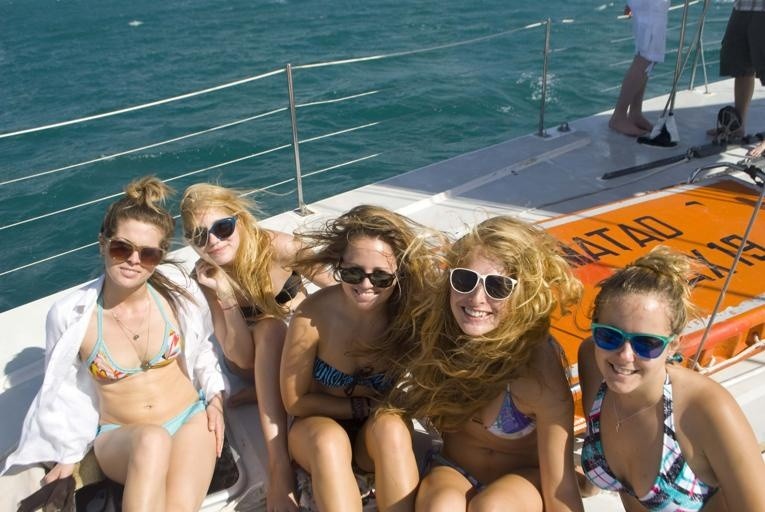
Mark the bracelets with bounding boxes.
[222,303,238,312]
[351,395,373,419]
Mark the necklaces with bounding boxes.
[103,289,154,373]
[606,385,666,434]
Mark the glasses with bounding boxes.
[591,322,679,359]
[103,236,166,267]
[448,267,516,300]
[339,260,399,287]
[185,218,238,246]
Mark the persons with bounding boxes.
[610,0,670,138]
[413,214,590,512]
[0,176,231,511]
[181,181,342,512]
[574,245,764,511]
[277,205,444,512]
[706,1,765,159]
